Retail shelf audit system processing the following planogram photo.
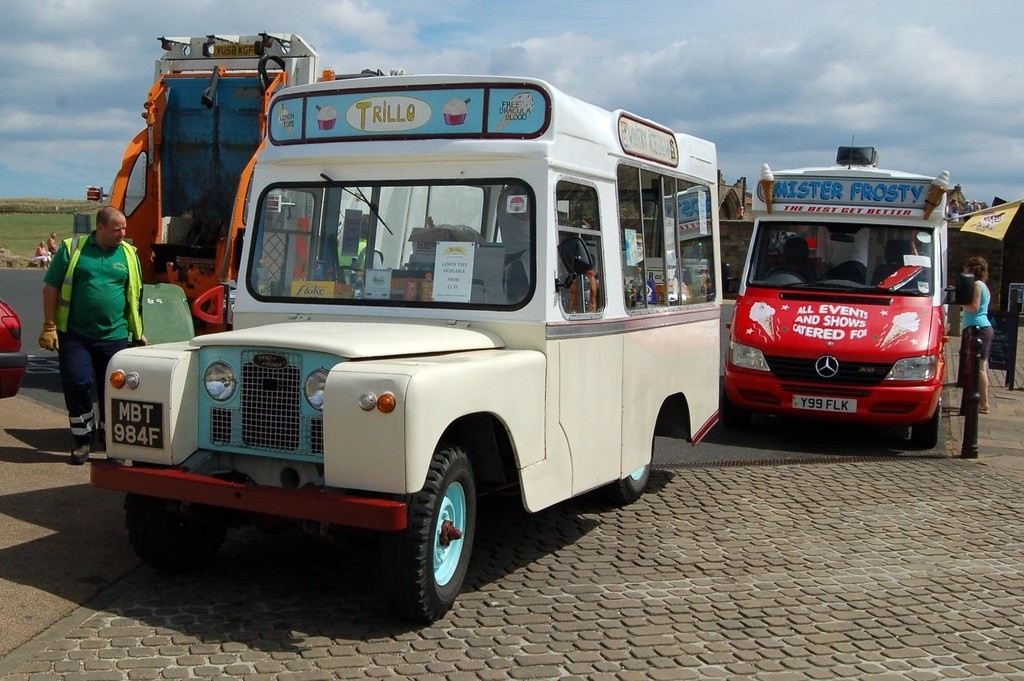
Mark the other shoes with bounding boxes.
[978,406,989,413]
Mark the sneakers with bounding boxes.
[71,435,92,463]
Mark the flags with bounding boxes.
[959,201,1021,240]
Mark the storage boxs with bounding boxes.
[407,227,452,262]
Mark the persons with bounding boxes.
[962,257,993,413]
[963,199,973,222]
[951,200,959,222]
[47,232,57,257]
[972,200,981,211]
[39,205,148,463]
[34,240,51,268]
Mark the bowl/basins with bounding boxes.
[444,113,466,125]
[319,119,336,130]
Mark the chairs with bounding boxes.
[767,236,811,286]
[870,238,926,292]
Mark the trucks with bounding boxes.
[103,26,407,344]
[722,139,979,453]
[89,71,721,625]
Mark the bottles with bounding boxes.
[421,272,433,302]
[271,279,284,296]
[403,277,417,301]
[562,272,601,315]
[681,267,714,305]
[257,258,269,296]
[340,257,365,298]
[405,256,432,295]
[314,260,326,281]
[622,265,645,309]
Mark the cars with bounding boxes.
[0,297,27,399]
